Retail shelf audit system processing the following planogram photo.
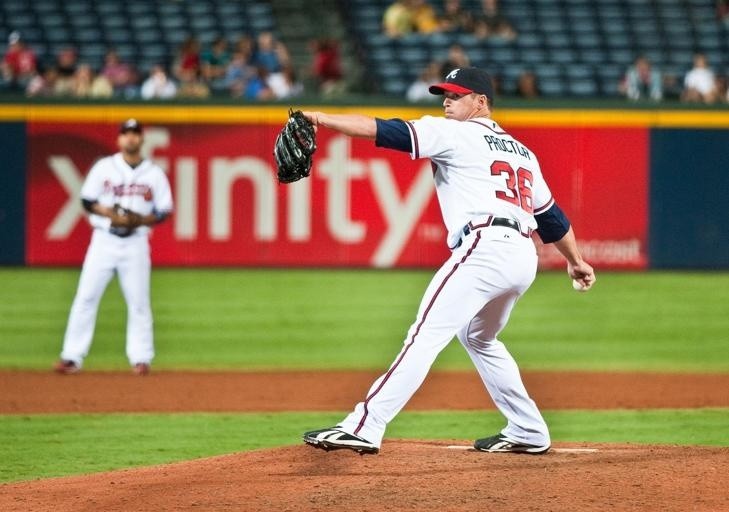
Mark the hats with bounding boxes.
[429,67,493,98]
[121,119,142,133]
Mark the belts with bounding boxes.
[457,217,518,247]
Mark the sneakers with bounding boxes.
[475,433,550,454]
[135,363,147,375]
[55,361,78,374]
[302,426,380,455]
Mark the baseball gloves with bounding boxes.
[108,203,141,236]
[273,107,316,184]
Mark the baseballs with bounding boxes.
[572,276,588,292]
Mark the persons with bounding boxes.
[621,57,674,101]
[289,66,596,453]
[680,51,729,104]
[50,118,174,376]
[0,30,345,101]
[383,0,544,102]
